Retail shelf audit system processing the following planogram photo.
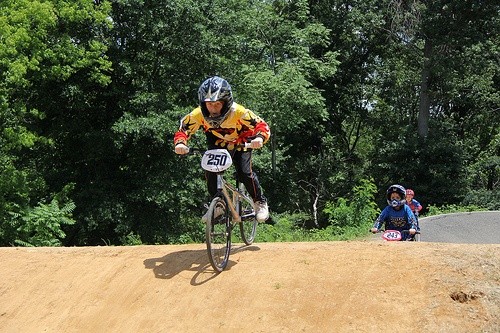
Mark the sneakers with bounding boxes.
[202,201,225,222]
[255,197,269,222]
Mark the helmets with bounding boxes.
[198,77,232,124]
[407,189,414,196]
[386,185,407,207]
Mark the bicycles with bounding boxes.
[369,229,420,241]
[189,142,258,273]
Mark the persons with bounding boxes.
[174,76,271,222]
[405,189,422,241]
[371,184,417,241]
